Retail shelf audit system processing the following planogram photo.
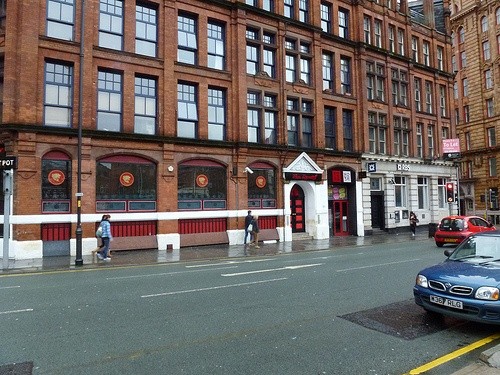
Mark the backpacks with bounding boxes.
[95,226,104,238]
[246,224,253,232]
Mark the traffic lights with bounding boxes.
[446,182,455,203]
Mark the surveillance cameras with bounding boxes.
[243,167,253,174]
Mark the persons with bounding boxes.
[244,210,253,245]
[91,215,112,261]
[410,211,417,240]
[248,215,261,249]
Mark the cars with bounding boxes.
[412,230,500,325]
[434,216,496,247]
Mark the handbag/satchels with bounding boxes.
[414,218,419,223]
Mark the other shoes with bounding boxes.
[412,233,416,237]
[243,242,261,249]
[92,249,112,260]
